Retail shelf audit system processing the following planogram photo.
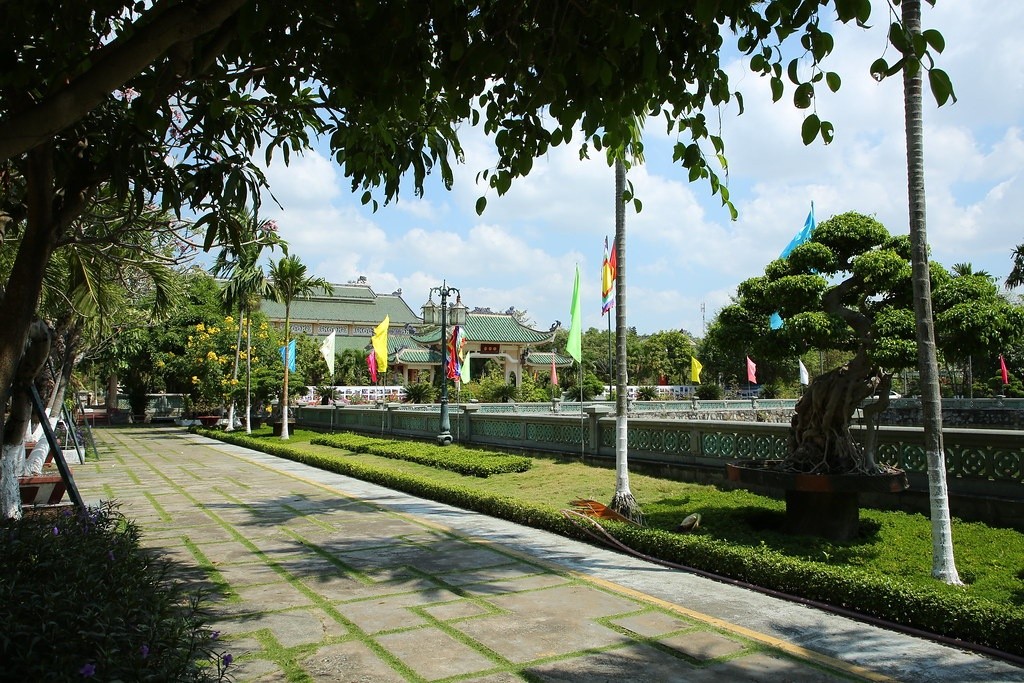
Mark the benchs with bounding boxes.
[78,413,111,428]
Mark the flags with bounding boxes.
[319,330,335,376]
[366,350,377,383]
[443,326,471,385]
[746,356,757,384]
[599,239,616,316]
[769,205,820,331]
[550,351,559,385]
[278,339,296,373]
[691,356,703,385]
[371,316,389,373]
[609,236,617,280]
[798,359,810,385]
[999,355,1008,385]
[566,263,583,363]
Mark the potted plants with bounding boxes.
[235,387,267,428]
[699,209,1024,541]
[256,361,308,435]
[194,402,224,427]
[173,393,230,426]
[0,237,70,502]
